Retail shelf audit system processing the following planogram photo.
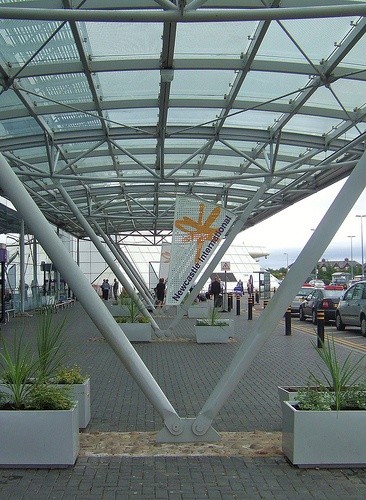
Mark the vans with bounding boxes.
[335,280,366,336]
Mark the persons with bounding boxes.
[101,279,111,301]
[18,280,50,302]
[247,279,252,297]
[237,280,245,295]
[189,277,224,307]
[113,279,119,300]
[155,278,165,310]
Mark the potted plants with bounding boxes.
[278,323,366,469]
[110,288,152,343]
[0,305,91,469]
[194,296,234,343]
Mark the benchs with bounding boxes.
[0,309,15,322]
[56,299,73,315]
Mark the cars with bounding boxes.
[290,287,317,313]
[299,285,347,324]
[308,271,366,288]
[234,287,244,296]
